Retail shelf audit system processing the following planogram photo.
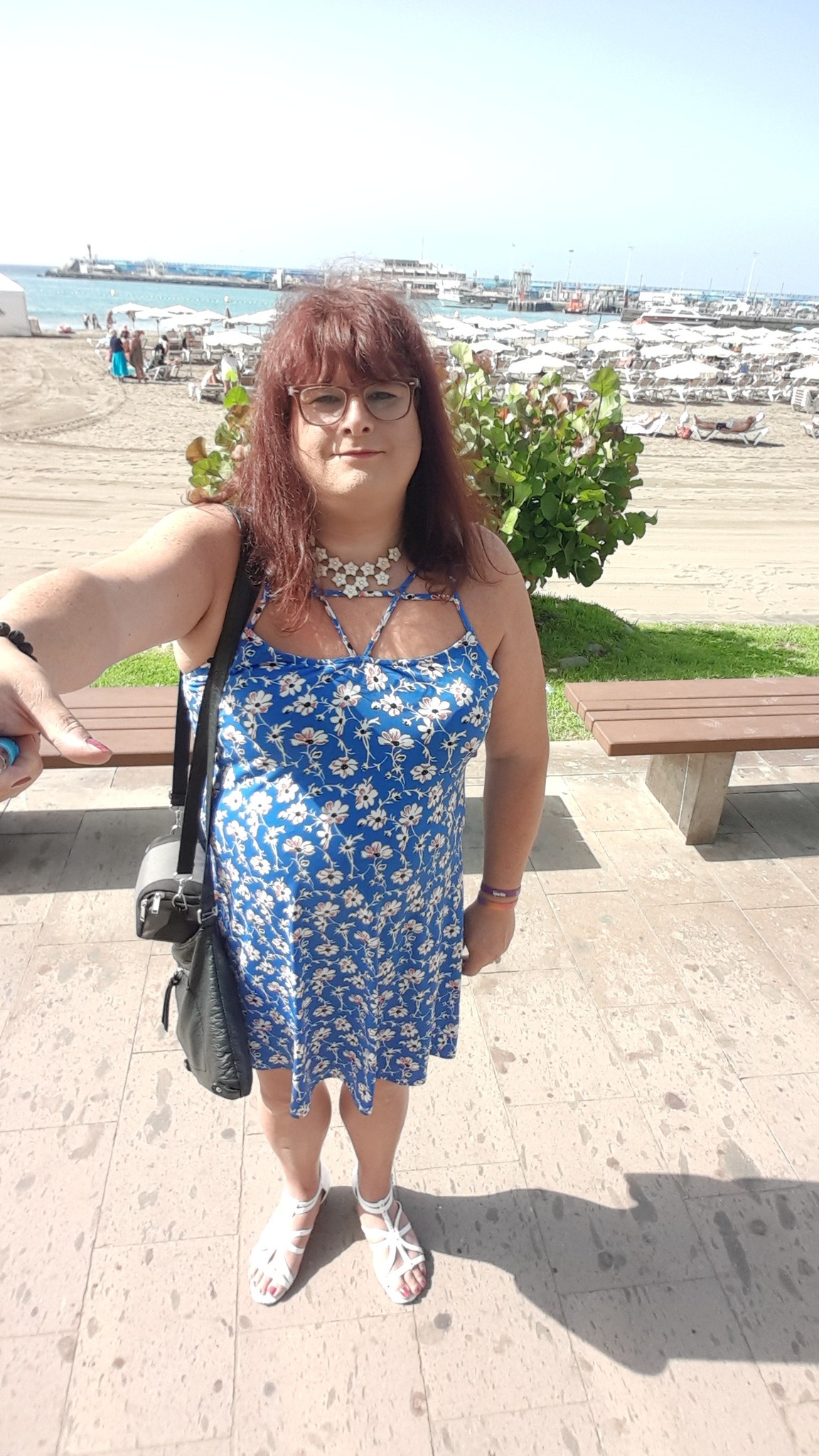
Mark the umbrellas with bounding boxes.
[111,303,279,358]
[415,312,819,387]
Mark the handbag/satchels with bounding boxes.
[161,904,253,1099]
[135,827,208,944]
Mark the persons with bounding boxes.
[81,312,242,396]
[692,415,756,431]
[0,281,551,1308]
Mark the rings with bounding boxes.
[495,957,501,964]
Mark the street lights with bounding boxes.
[623,246,633,296]
[566,250,574,290]
[745,252,759,298]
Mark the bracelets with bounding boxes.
[0,622,38,662]
[480,882,521,897]
[477,892,517,909]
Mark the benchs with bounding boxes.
[39,687,195,768]
[563,675,819,844]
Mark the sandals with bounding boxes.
[351,1161,426,1304]
[250,1160,331,1305]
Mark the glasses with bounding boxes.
[288,377,419,426]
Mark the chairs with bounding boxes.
[91,322,819,445]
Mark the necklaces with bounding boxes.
[307,534,404,599]
